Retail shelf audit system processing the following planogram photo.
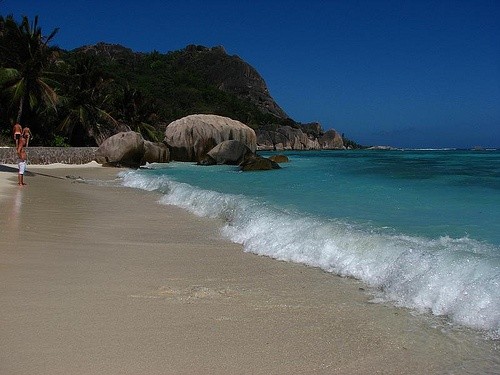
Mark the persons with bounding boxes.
[18,138,28,186]
[23,125,35,148]
[15,121,23,146]
